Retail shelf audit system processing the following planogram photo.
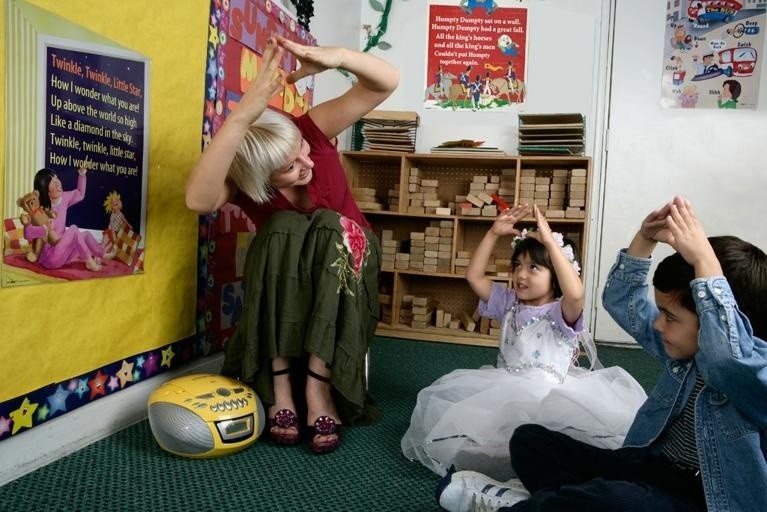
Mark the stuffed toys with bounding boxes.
[16,189,62,263]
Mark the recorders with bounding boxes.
[147,372,267,459]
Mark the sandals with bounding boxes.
[266,367,302,447]
[305,367,343,455]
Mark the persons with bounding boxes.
[23,156,116,272]
[400,199,651,483]
[182,36,404,454]
[436,196,765,511]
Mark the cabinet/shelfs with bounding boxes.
[337,150,595,346]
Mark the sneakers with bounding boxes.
[434,469,531,511]
